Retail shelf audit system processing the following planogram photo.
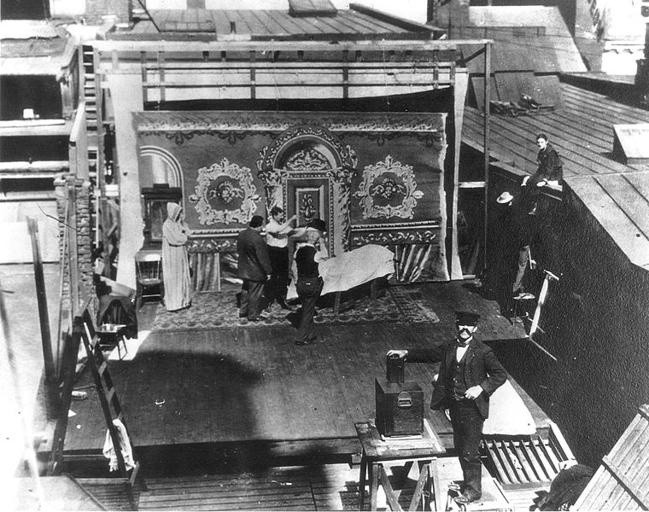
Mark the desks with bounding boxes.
[354,419,445,512]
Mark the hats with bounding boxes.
[496,191,515,204]
[452,310,483,327]
[306,218,327,232]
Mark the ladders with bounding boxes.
[46,296,148,511]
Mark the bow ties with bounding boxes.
[458,342,466,347]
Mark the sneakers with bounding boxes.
[239,298,319,346]
[448,479,484,506]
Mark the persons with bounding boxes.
[387,310,507,505]
[159,201,192,311]
[290,219,329,345]
[495,191,536,317]
[236,215,272,321]
[265,206,297,312]
[525,133,562,217]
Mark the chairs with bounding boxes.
[135,254,163,309]
